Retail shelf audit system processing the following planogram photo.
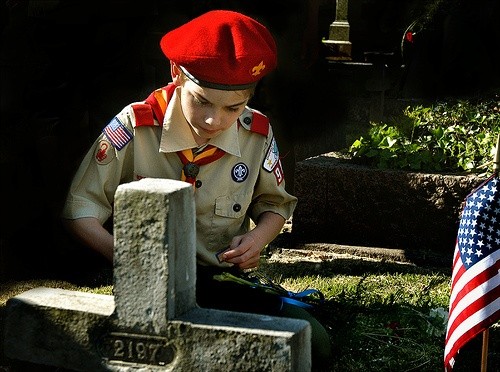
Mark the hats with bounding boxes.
[159,10,277,91]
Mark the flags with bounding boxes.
[444,174,500,372]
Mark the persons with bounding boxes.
[61,9,335,372]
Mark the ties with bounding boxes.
[145,82,240,197]
